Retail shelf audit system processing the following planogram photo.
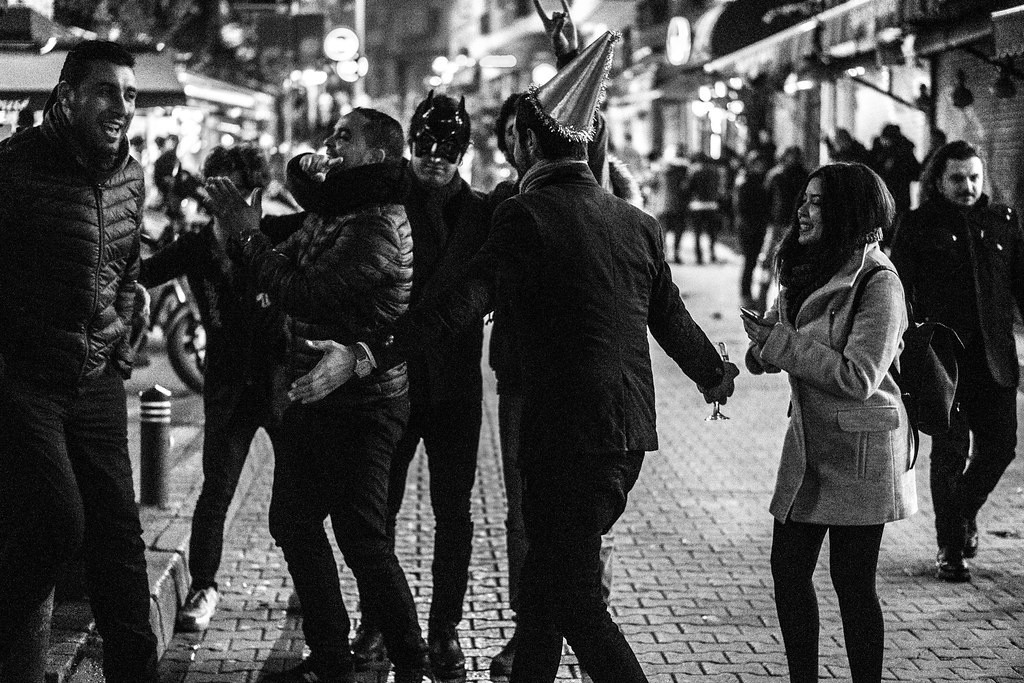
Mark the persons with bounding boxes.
[741,164,918,683]
[132,0,739,683]
[0,40,162,683]
[892,142,1024,582]
[641,123,946,266]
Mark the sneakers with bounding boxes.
[349,621,389,671]
[487,623,519,682]
[181,586,217,632]
[426,622,465,683]
[262,649,353,683]
[388,635,434,681]
[289,586,302,608]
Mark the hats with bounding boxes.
[532,31,621,142]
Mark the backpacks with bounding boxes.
[856,268,963,436]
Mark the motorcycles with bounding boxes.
[140,135,216,393]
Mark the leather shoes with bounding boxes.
[938,544,973,581]
[964,516,980,557]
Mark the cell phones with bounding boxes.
[741,307,760,326]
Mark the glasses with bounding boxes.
[415,130,461,162]
